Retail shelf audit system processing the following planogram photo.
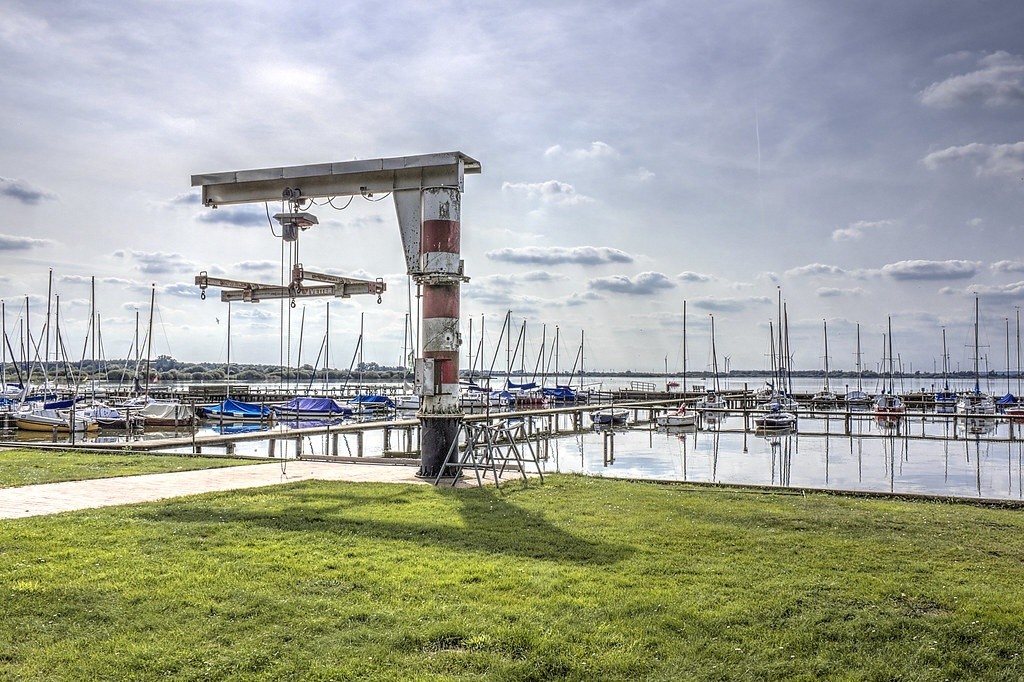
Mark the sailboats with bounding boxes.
[0,268,1024,452]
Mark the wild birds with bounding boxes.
[215,317,220,324]
